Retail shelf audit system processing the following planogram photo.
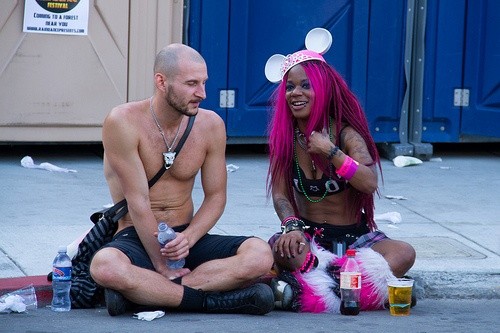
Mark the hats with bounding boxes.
[265,27,332,84]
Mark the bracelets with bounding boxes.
[326,145,340,161]
[281,215,311,234]
[335,155,360,182]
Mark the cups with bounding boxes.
[0,283,38,311]
[386,278,415,317]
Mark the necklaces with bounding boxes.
[149,95,185,170]
[292,116,333,203]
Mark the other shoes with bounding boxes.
[275,279,301,312]
[402,276,417,307]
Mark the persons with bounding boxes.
[90,42,276,316]
[266,50,416,312]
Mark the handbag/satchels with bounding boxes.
[90,207,119,240]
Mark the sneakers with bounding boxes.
[105,287,159,317]
[199,283,274,315]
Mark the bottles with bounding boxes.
[157,222,185,269]
[51,246,72,312]
[339,250,361,315]
